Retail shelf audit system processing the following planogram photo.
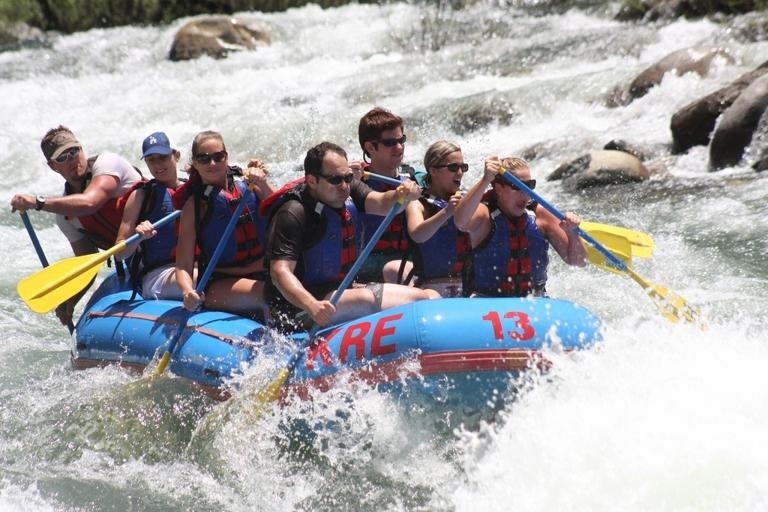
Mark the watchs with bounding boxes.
[34,193,46,211]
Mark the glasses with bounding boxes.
[433,163,468,172]
[53,147,81,165]
[501,180,536,191]
[371,135,406,147]
[196,151,227,164]
[319,173,353,185]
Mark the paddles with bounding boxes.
[362,172,653,277]
[16,209,181,314]
[499,167,701,326]
[256,195,405,405]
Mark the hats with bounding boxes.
[140,128,177,161]
[41,131,80,163]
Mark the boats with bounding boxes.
[68,265,609,437]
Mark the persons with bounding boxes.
[405,142,468,281]
[349,107,426,287]
[257,139,429,332]
[10,123,145,327]
[454,155,589,297]
[114,129,270,302]
